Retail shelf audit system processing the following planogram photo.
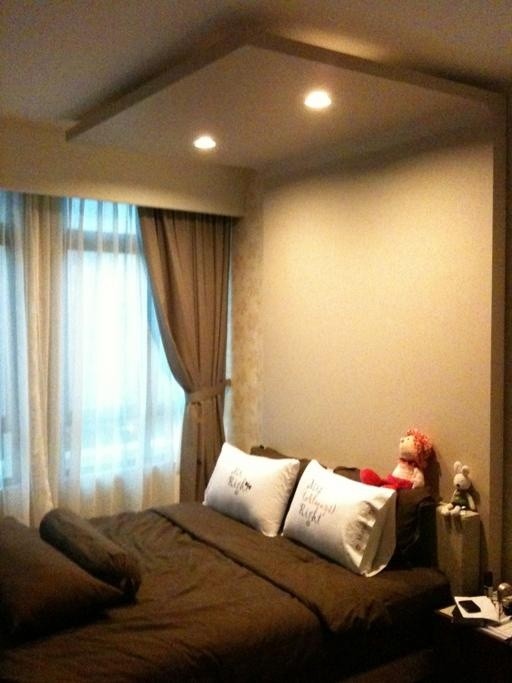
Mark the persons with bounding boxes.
[360,428,432,489]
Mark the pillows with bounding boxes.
[280,456,396,578]
[247,444,328,535]
[38,499,146,595]
[202,442,300,539]
[1,511,134,655]
[332,464,436,573]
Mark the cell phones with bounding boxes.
[458,600,481,613]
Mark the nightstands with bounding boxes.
[433,598,510,683]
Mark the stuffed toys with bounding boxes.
[440,461,476,517]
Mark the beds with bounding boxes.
[1,443,456,683]
